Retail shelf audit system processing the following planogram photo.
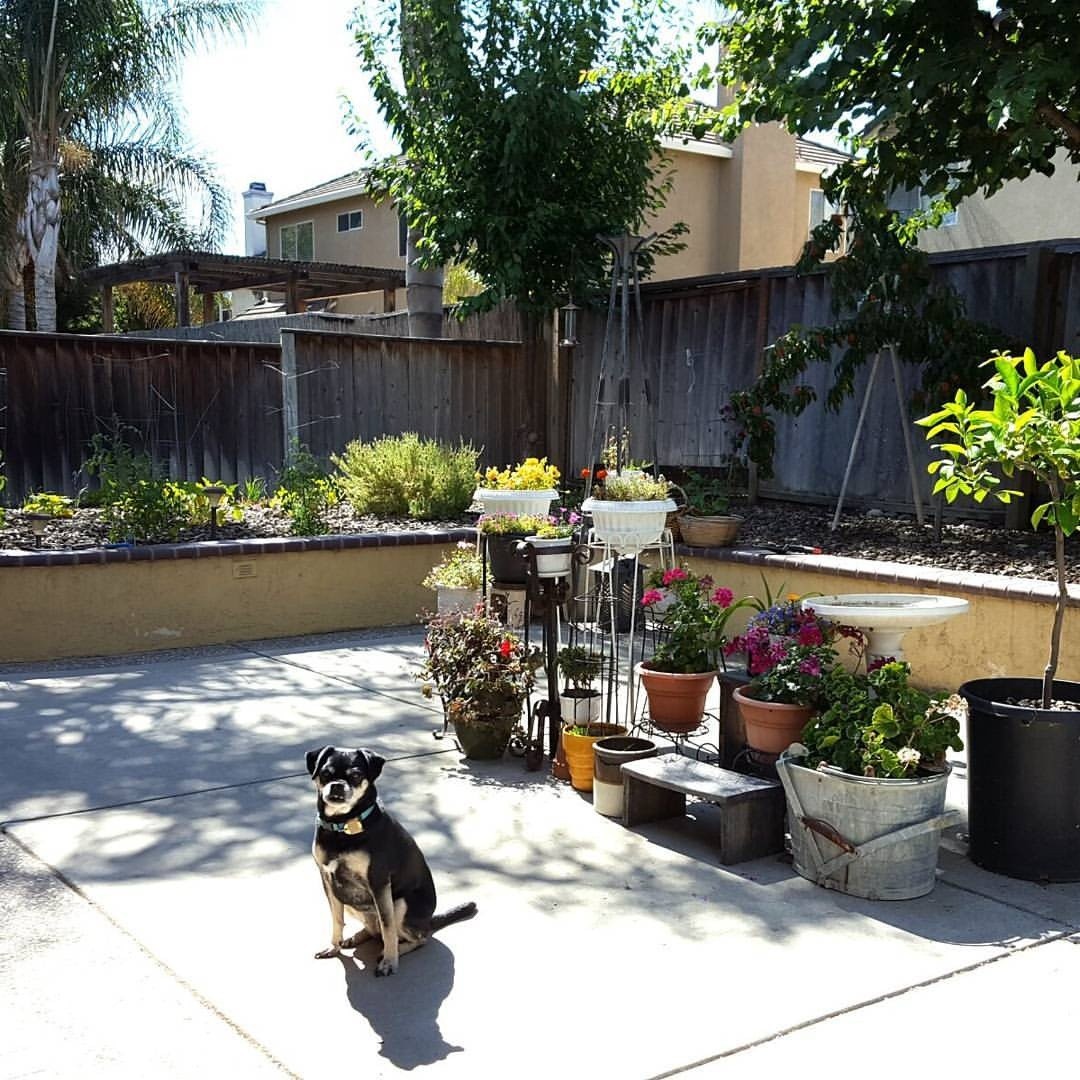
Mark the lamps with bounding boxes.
[24,512,51,534]
[588,553,649,635]
[561,291,583,346]
[202,487,226,507]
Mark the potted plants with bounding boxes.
[675,464,744,545]
[552,648,629,794]
[912,347,1080,884]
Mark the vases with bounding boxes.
[491,586,529,628]
[524,535,571,574]
[590,664,817,821]
[436,584,482,617]
[582,494,679,554]
[775,748,960,899]
[451,673,521,759]
[473,488,559,520]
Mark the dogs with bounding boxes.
[303,745,477,978]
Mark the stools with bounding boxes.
[621,751,785,866]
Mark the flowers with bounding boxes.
[640,560,965,778]
[409,458,580,730]
[580,430,672,502]
